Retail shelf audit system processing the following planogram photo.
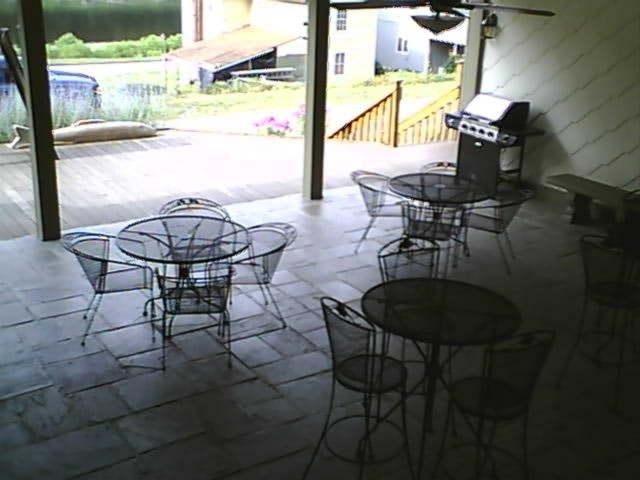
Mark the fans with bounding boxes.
[329,0,556,34]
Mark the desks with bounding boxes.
[546,172,639,225]
[592,221,639,357]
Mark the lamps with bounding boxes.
[480,12,498,39]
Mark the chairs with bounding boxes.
[554,233,639,415]
